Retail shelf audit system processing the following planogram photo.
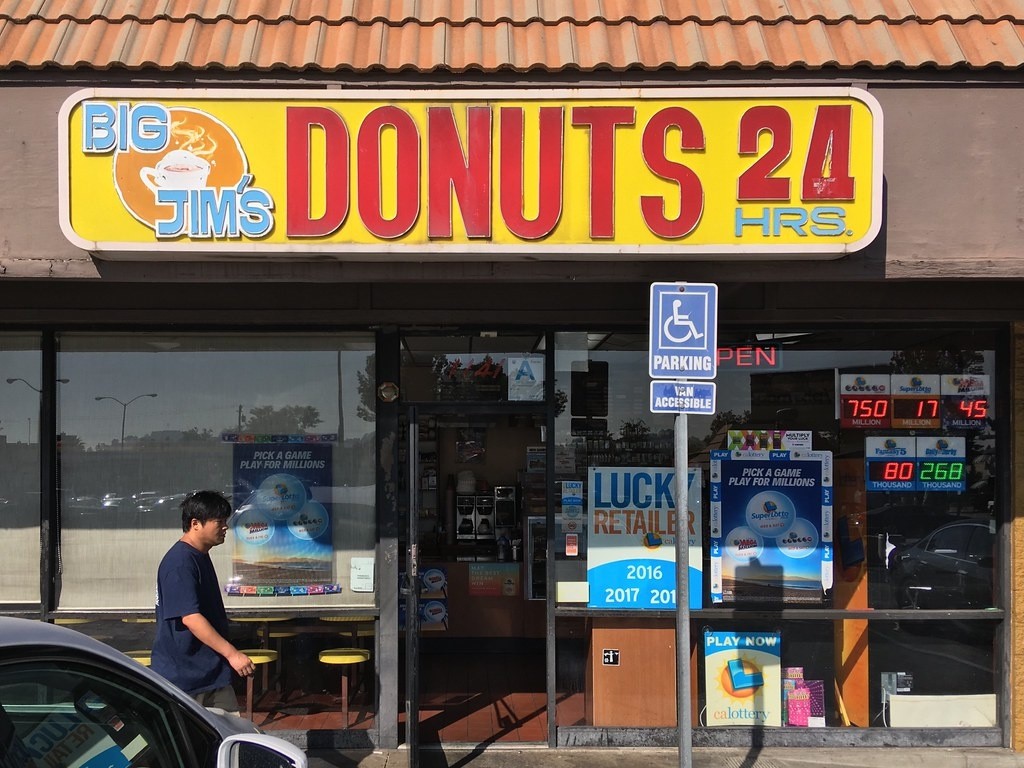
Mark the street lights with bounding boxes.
[238,405,245,431]
[94,393,157,475]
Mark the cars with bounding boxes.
[888,516,996,642]
[0,616,306,768]
[67,490,186,518]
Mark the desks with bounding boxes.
[29,616,375,704]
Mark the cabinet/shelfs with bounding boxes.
[516,434,674,600]
[396,416,446,558]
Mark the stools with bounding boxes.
[89,624,376,731]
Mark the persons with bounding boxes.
[151,488,256,716]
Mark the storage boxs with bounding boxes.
[781,666,826,728]
